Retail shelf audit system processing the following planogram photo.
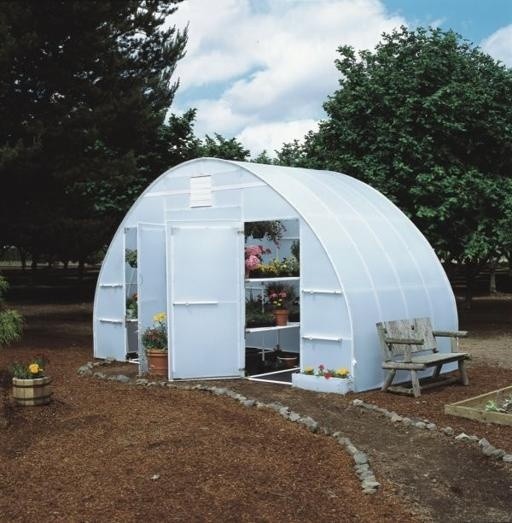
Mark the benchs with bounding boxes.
[376,317,473,399]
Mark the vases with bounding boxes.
[246,347,258,371]
[272,309,289,326]
[282,352,296,369]
[291,373,353,395]
[12,374,50,406]
[146,348,168,380]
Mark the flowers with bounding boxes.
[11,356,47,379]
[141,312,168,350]
[245,244,300,309]
[302,364,350,379]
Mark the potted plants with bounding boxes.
[125,248,137,268]
[245,308,276,328]
[245,221,287,249]
[126,298,137,319]
